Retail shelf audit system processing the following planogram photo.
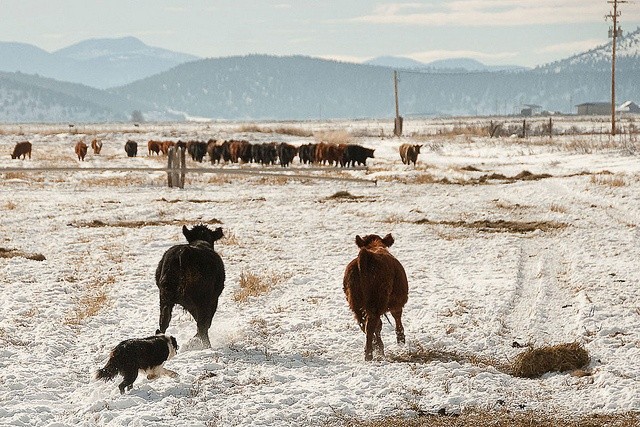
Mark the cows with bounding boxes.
[343,232,409,362]
[399,143,423,166]
[154,222,226,349]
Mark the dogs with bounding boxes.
[95,328,180,397]
